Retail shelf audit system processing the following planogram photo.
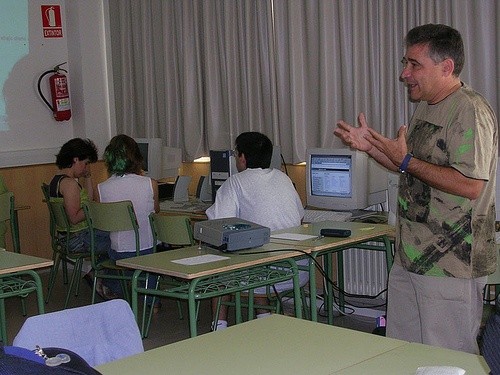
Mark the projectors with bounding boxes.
[193,218,270,251]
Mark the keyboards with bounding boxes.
[302,210,352,224]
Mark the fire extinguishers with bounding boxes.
[38,62,72,122]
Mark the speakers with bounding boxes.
[172,176,190,202]
[195,176,208,200]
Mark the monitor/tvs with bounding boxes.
[305,147,388,211]
[136,138,182,179]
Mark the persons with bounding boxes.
[50,137,119,301]
[94,134,161,312]
[336,23,498,356]
[212,132,310,331]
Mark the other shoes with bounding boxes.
[146,305,157,315]
[84,274,118,300]
[210,320,227,331]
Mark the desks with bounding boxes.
[113,240,312,340]
[258,218,395,324]
[156,202,391,225]
[0,246,55,346]
[94,312,489,375]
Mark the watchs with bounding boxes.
[398,153,414,175]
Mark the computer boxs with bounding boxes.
[209,149,238,202]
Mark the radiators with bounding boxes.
[333,241,393,301]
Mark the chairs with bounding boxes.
[0,191,29,317]
[210,265,309,328]
[82,198,183,335]
[10,299,146,365]
[147,212,197,340]
[39,182,82,297]
[44,196,104,310]
